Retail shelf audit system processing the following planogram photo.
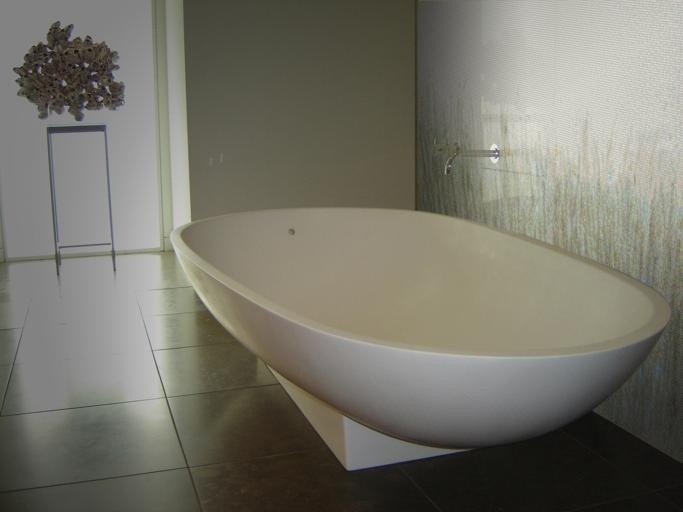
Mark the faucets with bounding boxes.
[443,138,498,176]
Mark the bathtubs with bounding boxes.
[169,207,672,472]
